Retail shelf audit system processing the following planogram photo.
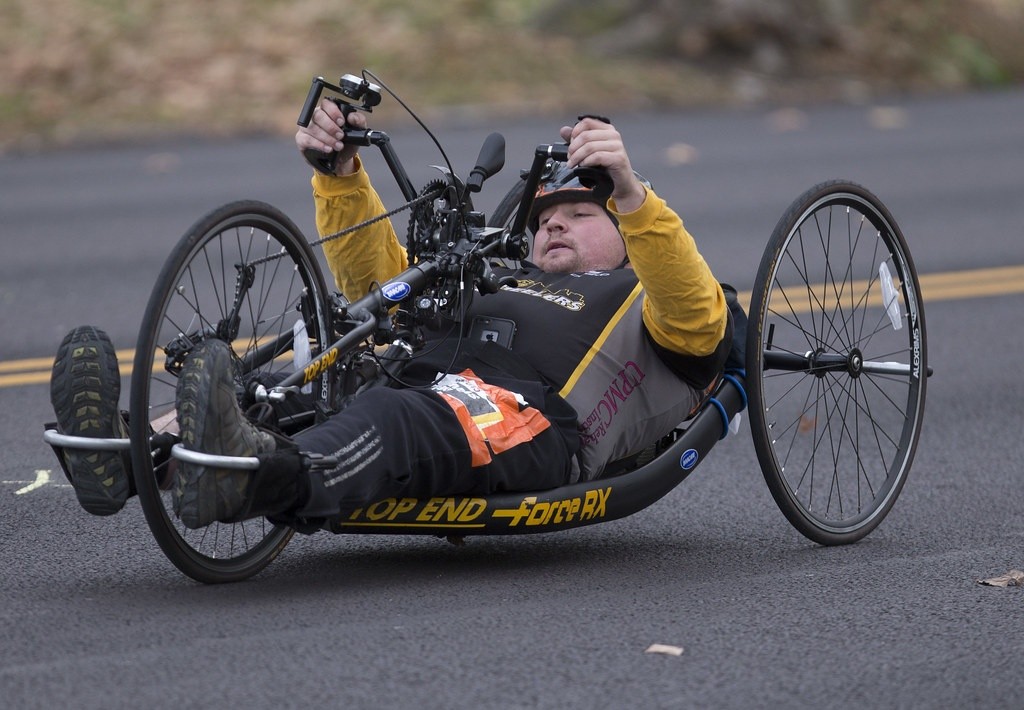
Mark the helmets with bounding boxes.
[524,157,654,240]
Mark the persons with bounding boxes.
[48,84,737,529]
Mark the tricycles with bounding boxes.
[39,69,933,587]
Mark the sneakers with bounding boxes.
[170,339,299,530]
[48,324,176,517]
